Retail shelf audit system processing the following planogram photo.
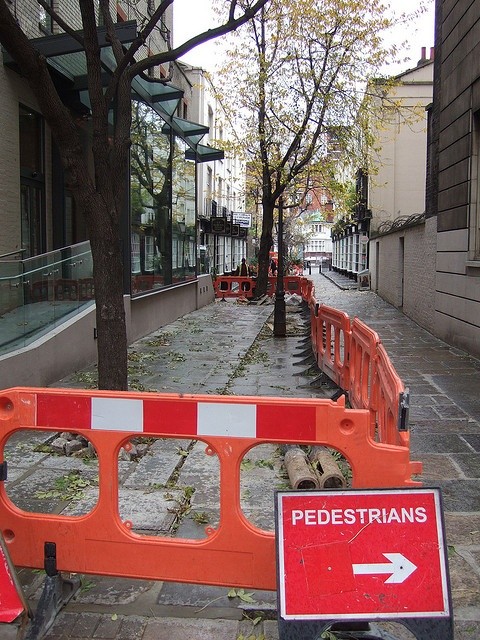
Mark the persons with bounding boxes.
[236,258,251,291]
[271,260,276,273]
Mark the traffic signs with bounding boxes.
[274,486,456,616]
